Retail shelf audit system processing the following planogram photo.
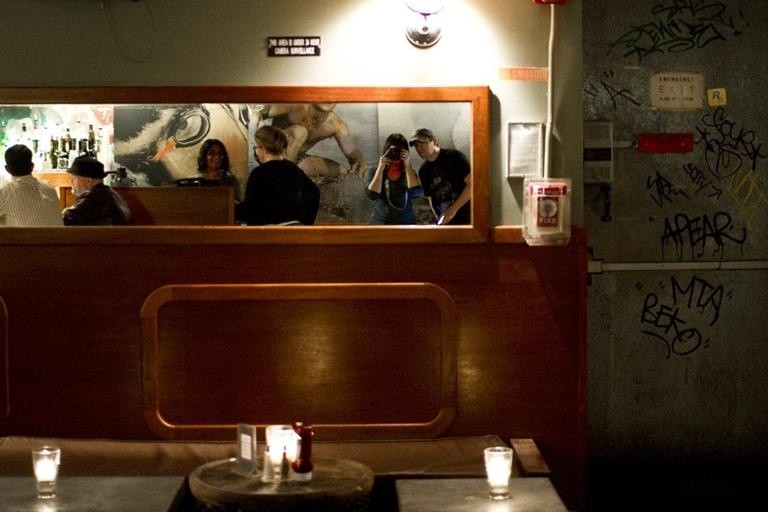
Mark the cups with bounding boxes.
[482,446,515,501]
[29,445,62,500]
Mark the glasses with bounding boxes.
[252,143,264,151]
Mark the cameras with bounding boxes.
[388,145,401,160]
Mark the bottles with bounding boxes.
[0,115,105,170]
[261,421,315,487]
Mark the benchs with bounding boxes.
[1,433,555,478]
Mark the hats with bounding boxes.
[65,156,109,179]
[409,128,434,148]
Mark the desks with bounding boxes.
[188,454,376,509]
[0,472,185,510]
[395,474,570,512]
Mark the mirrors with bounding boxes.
[1,83,492,250]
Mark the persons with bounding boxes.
[409,128,472,225]
[361,134,422,225]
[61,156,131,227]
[258,103,360,186]
[185,139,241,229]
[235,125,321,226]
[0,144,66,227]
